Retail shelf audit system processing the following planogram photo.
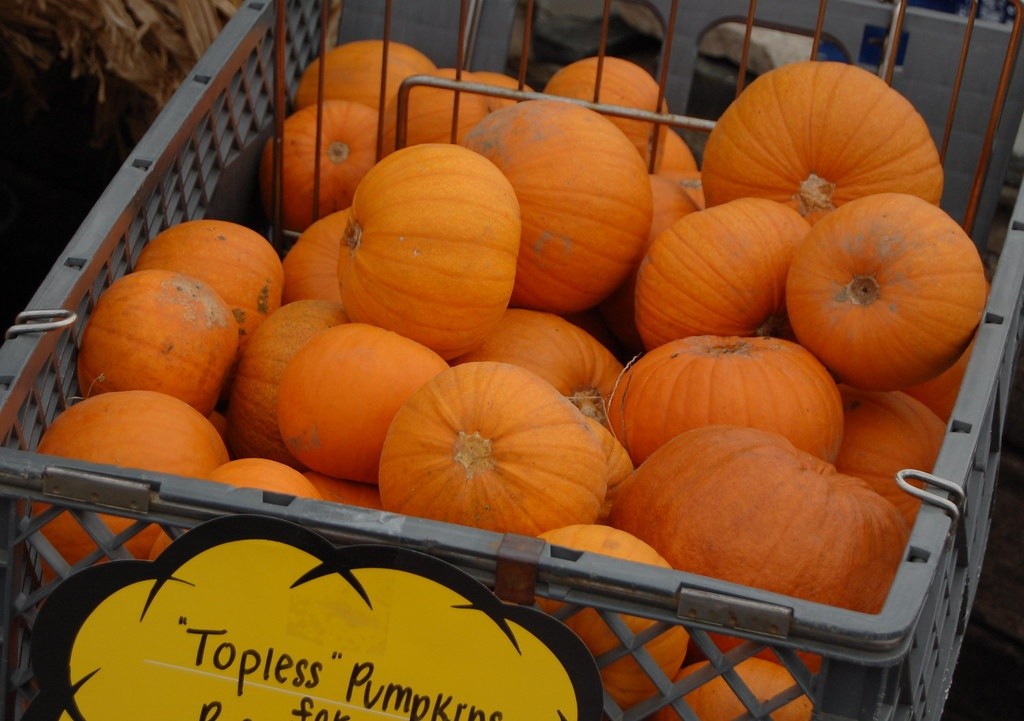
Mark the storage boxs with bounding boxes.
[0,0,1024,721]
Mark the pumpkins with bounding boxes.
[31,41,993,721]
